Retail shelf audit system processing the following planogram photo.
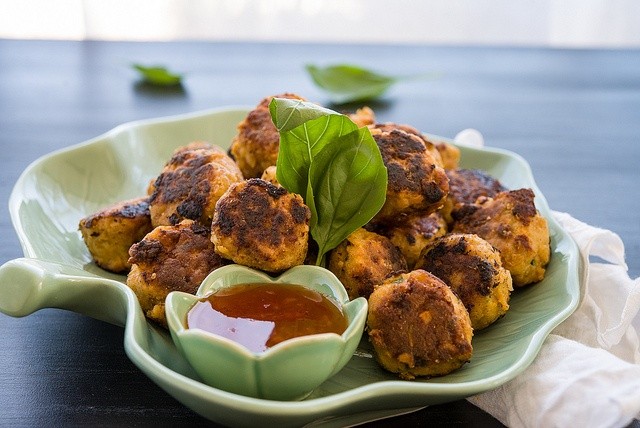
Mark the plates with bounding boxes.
[0,107,588,428]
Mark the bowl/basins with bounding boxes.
[164,264,369,403]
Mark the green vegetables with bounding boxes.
[304,125,389,268]
